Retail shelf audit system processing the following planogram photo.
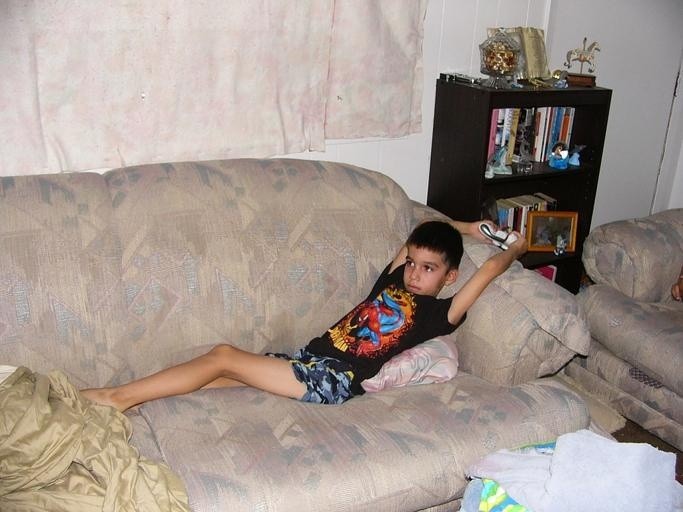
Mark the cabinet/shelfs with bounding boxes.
[427,69,612,297]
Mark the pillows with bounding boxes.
[359,333,460,391]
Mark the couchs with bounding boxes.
[572,206,681,456]
[0,157,592,512]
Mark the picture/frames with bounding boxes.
[526,211,578,252]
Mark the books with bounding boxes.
[533,264,559,283]
[483,104,576,248]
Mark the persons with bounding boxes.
[72,214,530,414]
